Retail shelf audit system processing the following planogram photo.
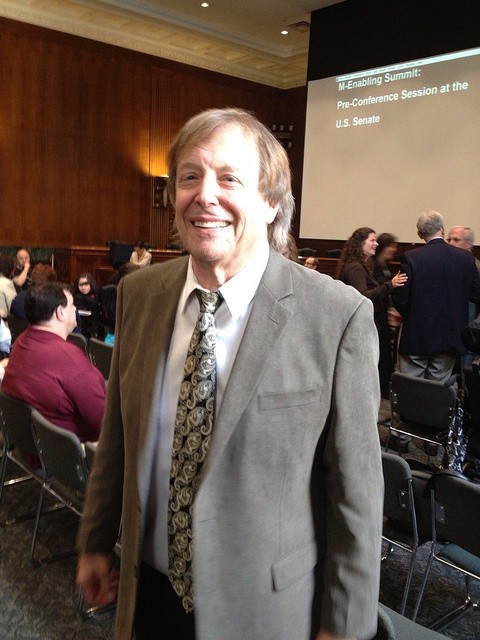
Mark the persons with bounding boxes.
[130,240,152,268]
[12,249,34,295]
[335,227,408,400]
[31,264,57,286]
[77,273,106,341]
[303,257,319,270]
[391,211,480,381]
[1,281,108,434]
[448,226,480,374]
[76,108,385,638]
[0,257,18,319]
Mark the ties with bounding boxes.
[166,288,225,615]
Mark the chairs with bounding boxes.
[368,600,448,635]
[0,390,74,526]
[166,244,183,251]
[144,243,156,249]
[28,410,89,561]
[84,441,96,469]
[382,370,458,472]
[297,247,319,257]
[106,240,118,247]
[381,452,471,629]
[326,249,342,258]
[89,337,113,381]
[66,333,91,364]
[411,468,480,639]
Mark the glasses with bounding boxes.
[80,282,89,287]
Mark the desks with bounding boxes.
[51,244,404,300]
